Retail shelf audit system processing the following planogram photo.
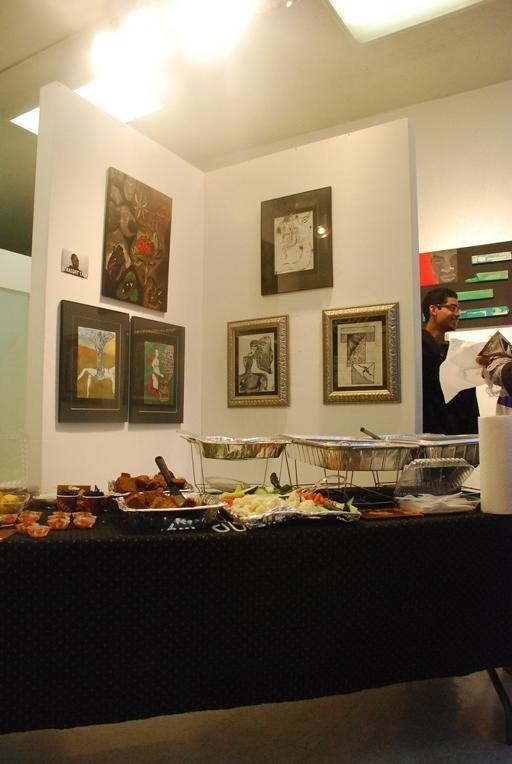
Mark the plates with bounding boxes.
[118,492,223,531]
[195,435,287,459]
[384,433,479,465]
[291,434,418,469]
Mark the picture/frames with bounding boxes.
[61,247,89,280]
[100,166,172,315]
[260,186,334,296]
[418,240,511,330]
[128,314,186,426]
[56,297,130,424]
[226,314,290,409]
[321,301,402,406]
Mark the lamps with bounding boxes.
[314,224,329,240]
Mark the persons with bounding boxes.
[482,344,512,414]
[419,287,481,435]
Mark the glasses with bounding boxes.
[435,302,460,311]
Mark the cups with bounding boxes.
[57,494,79,513]
[83,493,108,517]
